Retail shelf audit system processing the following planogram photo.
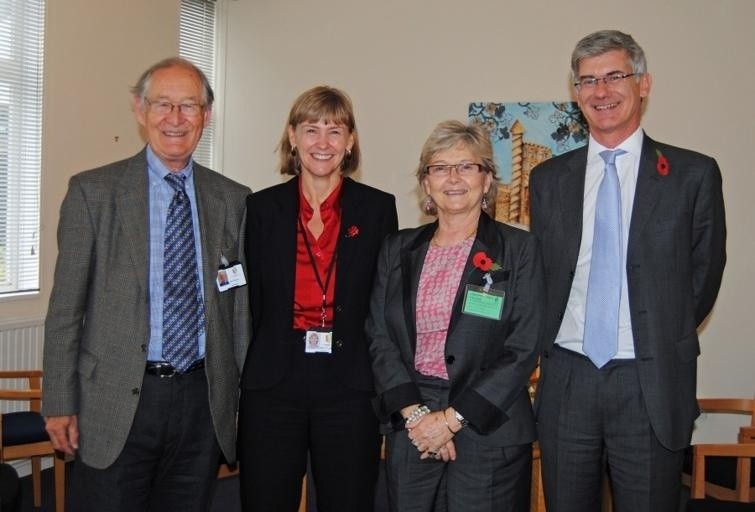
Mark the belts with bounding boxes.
[144,358,206,379]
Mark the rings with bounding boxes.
[426,447,437,456]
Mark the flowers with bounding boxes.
[469,248,504,273]
[346,223,359,240]
[652,146,670,178]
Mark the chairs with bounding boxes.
[684,443,755,511]
[680,397,755,501]
[0,370,67,511]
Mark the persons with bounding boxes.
[526,29,726,511]
[365,120,547,511]
[234,86,398,512]
[40,57,254,511]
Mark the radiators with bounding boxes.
[0,314,47,470]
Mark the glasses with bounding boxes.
[424,163,487,177]
[145,96,204,115]
[574,73,636,89]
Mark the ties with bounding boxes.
[582,148,628,370]
[162,172,206,375]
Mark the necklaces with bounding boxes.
[433,226,479,249]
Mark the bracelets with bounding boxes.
[401,403,431,432]
[454,411,467,429]
[443,409,456,436]
